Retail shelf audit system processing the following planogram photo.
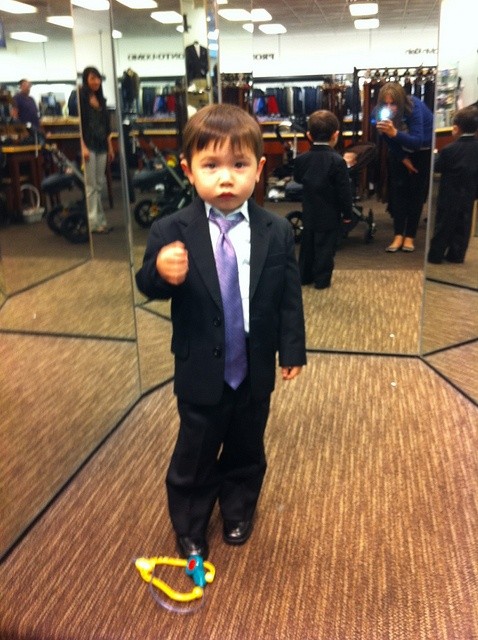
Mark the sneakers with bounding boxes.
[92,226,109,234]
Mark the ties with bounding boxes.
[207,208,247,391]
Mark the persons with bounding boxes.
[185,40,210,107]
[122,68,140,104]
[133,103,308,562]
[10,79,39,135]
[79,67,115,234]
[370,82,433,254]
[427,106,477,263]
[293,110,353,290]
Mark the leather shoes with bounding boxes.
[174,522,208,562]
[221,507,253,544]
[401,237,414,252]
[384,240,402,253]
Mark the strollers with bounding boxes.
[267,119,382,245]
[123,114,198,229]
[25,122,90,243]
